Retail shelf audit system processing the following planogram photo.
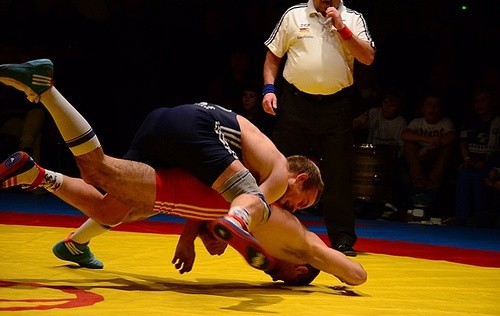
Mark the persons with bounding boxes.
[358,63,499,223]
[0,101,61,196]
[0,102,325,272]
[0,59,367,285]
[206,50,276,138]
[262,0,376,256]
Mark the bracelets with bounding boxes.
[337,24,352,41]
[262,84,275,95]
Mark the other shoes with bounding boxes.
[0,59,54,103]
[211,207,273,271]
[52,231,104,269]
[0,151,42,188]
[336,244,357,256]
